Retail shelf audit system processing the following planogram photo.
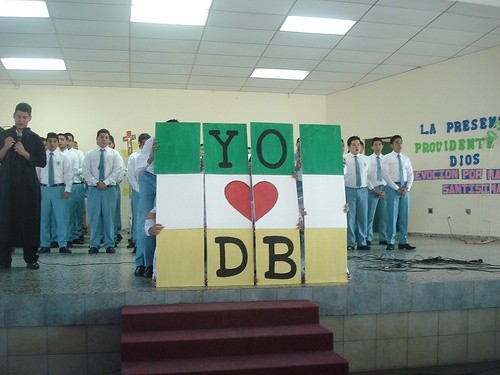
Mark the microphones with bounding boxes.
[17,132,23,143]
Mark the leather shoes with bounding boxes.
[398,244,416,250]
[379,241,387,244]
[39,248,50,253]
[88,247,98,254]
[348,246,353,250]
[27,261,39,270]
[106,247,115,252]
[357,246,371,250]
[387,245,394,250]
[144,266,152,277]
[367,241,371,245]
[60,247,72,253]
[134,266,145,276]
[48,228,135,248]
[133,248,136,253]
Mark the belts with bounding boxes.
[73,183,80,184]
[395,182,407,184]
[40,183,64,186]
[94,186,113,187]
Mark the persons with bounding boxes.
[381,135,416,250]
[360,142,364,154]
[36,132,74,254]
[367,137,388,246]
[199,137,351,286]
[0,103,47,274]
[82,129,123,253]
[99,119,182,288]
[40,132,88,248]
[344,136,371,250]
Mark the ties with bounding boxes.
[397,155,404,185]
[376,157,382,181]
[354,156,361,187]
[99,149,105,181]
[49,153,54,186]
